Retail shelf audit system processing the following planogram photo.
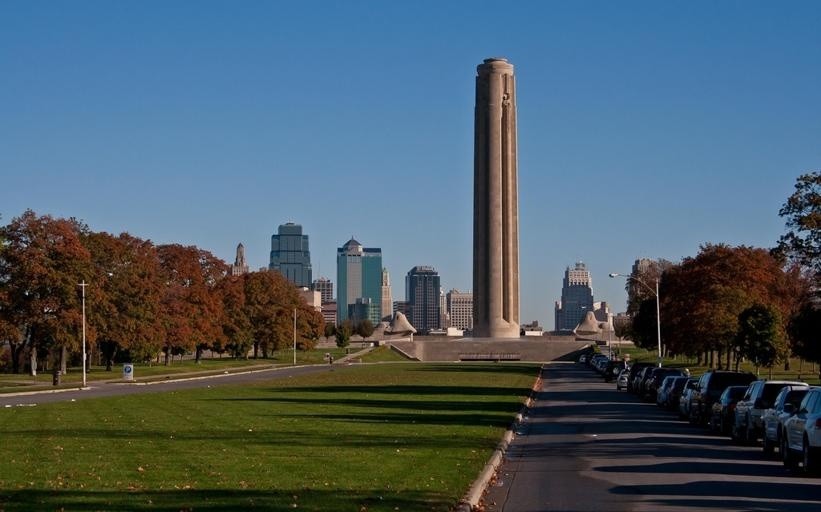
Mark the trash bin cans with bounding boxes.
[325,353,330,360]
[123,363,134,381]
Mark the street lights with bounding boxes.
[78,272,114,388]
[609,273,661,358]
[292,306,325,366]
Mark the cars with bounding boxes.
[579,353,821,473]
